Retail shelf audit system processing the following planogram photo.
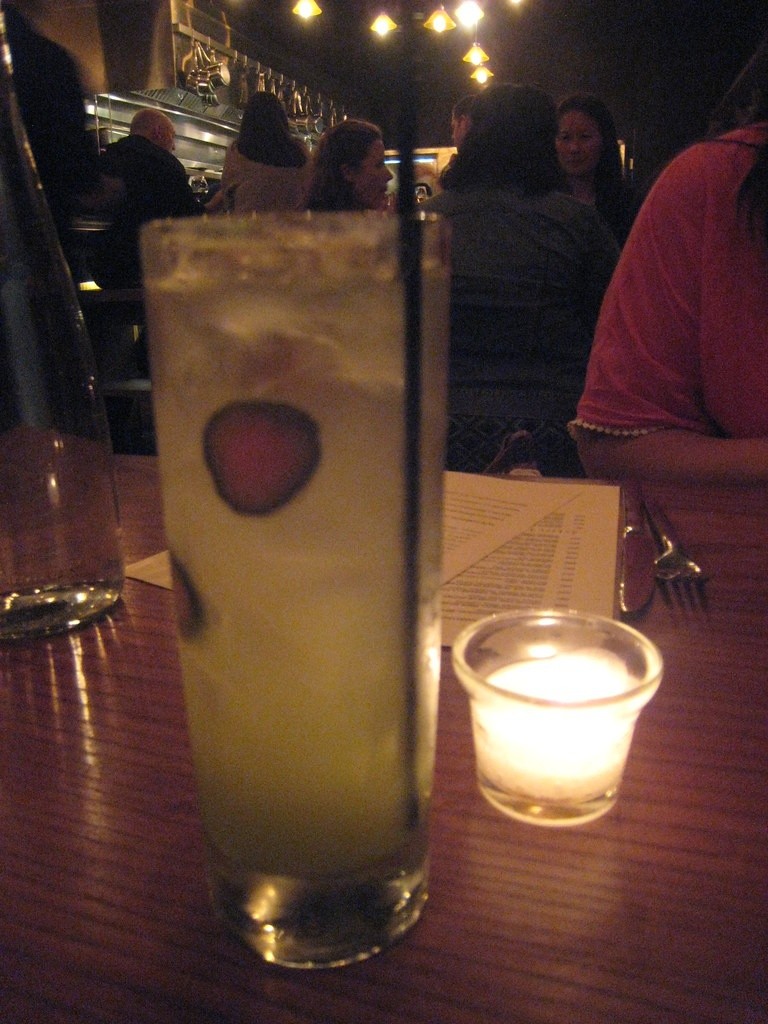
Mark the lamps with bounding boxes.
[290,0,494,86]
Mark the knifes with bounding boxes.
[620,480,656,614]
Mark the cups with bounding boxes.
[450,609,663,829]
[139,214,453,972]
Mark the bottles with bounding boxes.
[0,17,125,646]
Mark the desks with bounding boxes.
[0,424,768,1024]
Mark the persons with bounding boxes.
[418,86,639,482]
[568,40,767,484]
[92,112,220,374]
[298,119,391,211]
[0,0,128,440]
[220,93,309,211]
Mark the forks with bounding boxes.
[635,474,713,622]
